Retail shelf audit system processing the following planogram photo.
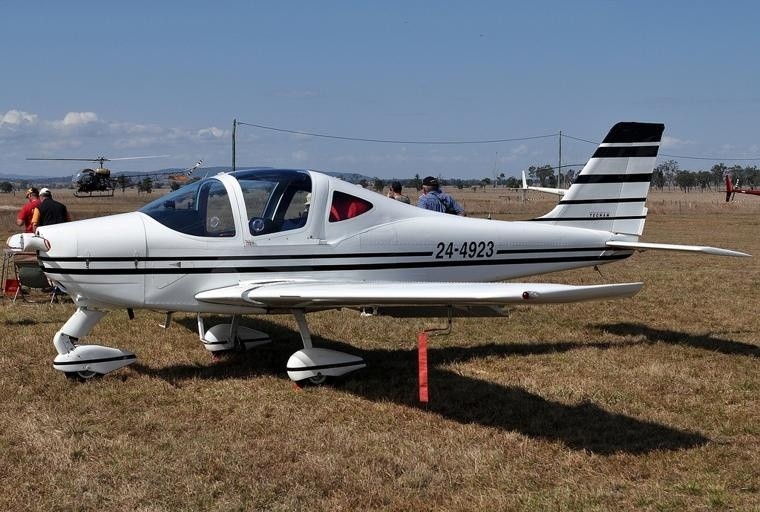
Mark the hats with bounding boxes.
[23,187,51,198]
[423,177,438,186]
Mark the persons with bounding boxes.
[16,187,71,233]
[186,197,196,209]
[389,176,465,218]
[305,192,311,207]
[725,174,732,202]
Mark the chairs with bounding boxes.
[11,253,66,304]
